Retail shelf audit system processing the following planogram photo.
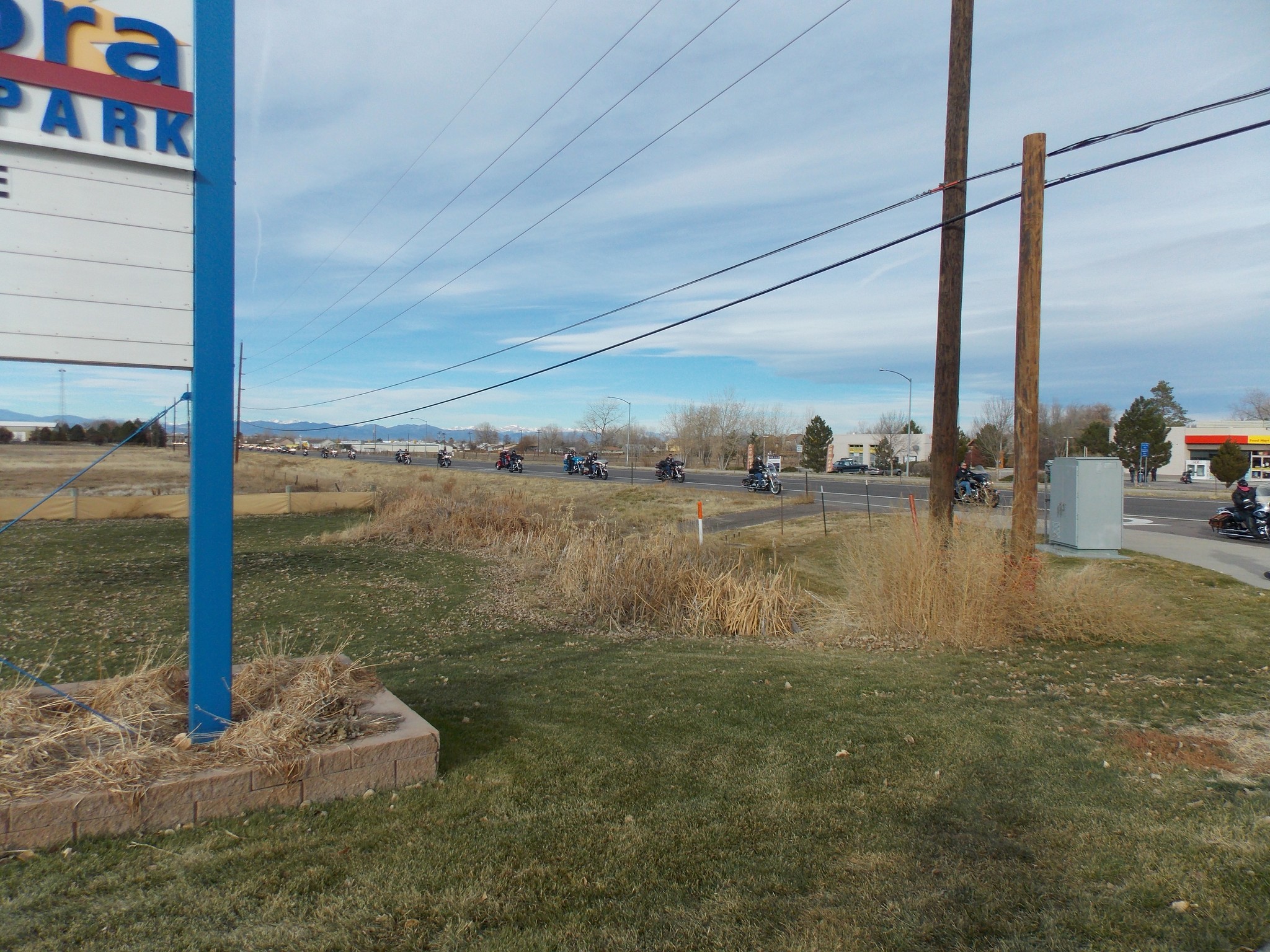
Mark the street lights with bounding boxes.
[410,417,427,458]
[763,435,769,465]
[968,450,972,468]
[607,395,631,466]
[879,368,912,476]
[1063,436,1074,458]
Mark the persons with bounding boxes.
[1232,479,1264,539]
[330,447,337,455]
[398,449,403,452]
[1138,465,1144,482]
[1150,466,1157,482]
[1143,465,1150,482]
[439,449,448,465]
[350,447,354,451]
[1129,463,1135,483]
[322,446,326,454]
[501,449,509,465]
[404,449,408,454]
[510,450,517,469]
[752,456,767,487]
[303,446,309,451]
[567,449,576,471]
[586,452,599,475]
[664,454,672,477]
[955,462,975,500]
[291,446,294,449]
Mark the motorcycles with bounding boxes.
[395,452,412,465]
[564,454,585,476]
[348,449,356,460]
[289,448,296,455]
[330,449,338,458]
[321,448,330,459]
[952,466,1001,507]
[585,456,608,480]
[1180,468,1193,484]
[495,454,524,473]
[655,460,686,483]
[302,449,309,456]
[238,444,282,452]
[437,451,452,468]
[741,461,783,494]
[1209,485,1270,543]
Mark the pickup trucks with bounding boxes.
[832,460,868,475]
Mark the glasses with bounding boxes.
[670,456,672,457]
[962,465,967,466]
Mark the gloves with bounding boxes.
[1245,502,1252,506]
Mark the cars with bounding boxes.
[868,464,903,476]
[281,446,291,454]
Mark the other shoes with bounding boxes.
[1255,534,1268,539]
[968,495,972,498]
[593,474,596,475]
[759,484,763,488]
[668,475,672,478]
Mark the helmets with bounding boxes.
[506,450,509,451]
[593,452,597,455]
[1238,479,1248,487]
[503,449,505,451]
[756,456,762,461]
[572,451,576,454]
[569,451,573,455]
[512,451,515,453]
[588,452,593,456]
